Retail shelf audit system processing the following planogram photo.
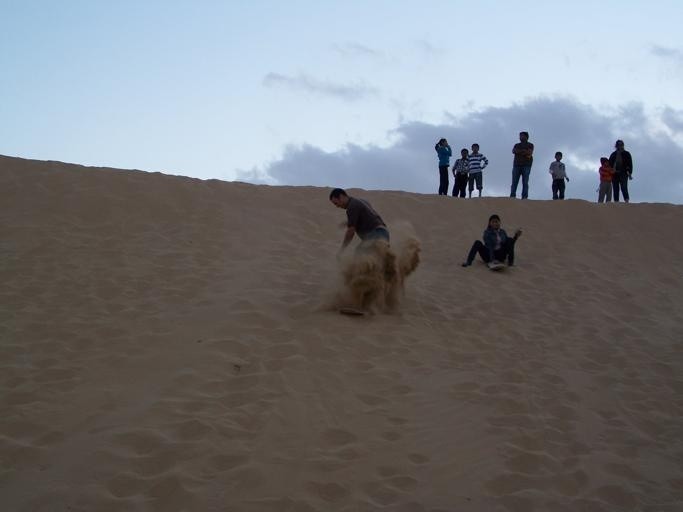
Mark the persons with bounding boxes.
[460,214,523,267]
[328,187,389,316]
[434,138,452,195]
[608,140,632,203]
[466,144,488,198]
[597,157,616,204]
[548,152,570,200]
[509,132,534,200]
[451,149,470,198]
